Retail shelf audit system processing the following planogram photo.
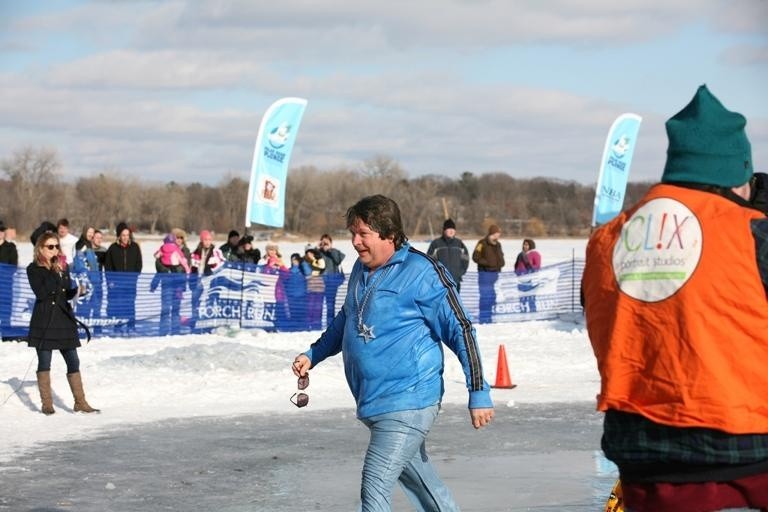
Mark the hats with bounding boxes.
[443,218,456,230]
[490,225,501,234]
[117,222,128,236]
[661,84,753,187]
[166,233,175,243]
[200,231,282,259]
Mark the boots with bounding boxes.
[66,372,98,412]
[38,371,55,414]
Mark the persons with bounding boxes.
[514,239,541,313]
[471,225,505,324]
[291,194,496,512]
[580,84,768,512]
[29,220,142,339]
[27,233,100,414]
[0,223,18,327]
[150,226,345,341]
[427,218,469,294]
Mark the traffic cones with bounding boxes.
[492,345,517,390]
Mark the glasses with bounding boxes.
[290,360,309,407]
[43,245,60,250]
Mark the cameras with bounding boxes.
[320,242,328,246]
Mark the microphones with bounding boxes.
[50,256,63,281]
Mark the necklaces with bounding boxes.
[354,267,391,344]
